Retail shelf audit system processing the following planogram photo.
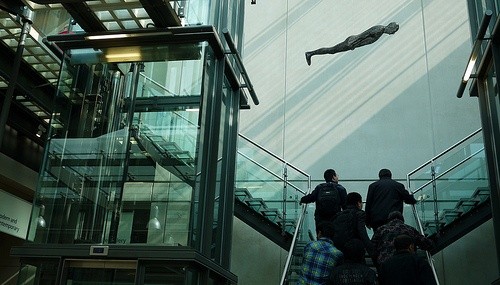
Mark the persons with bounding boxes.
[295,192,436,285]
[365,169,417,231]
[305,22,399,66]
[298,169,347,232]
